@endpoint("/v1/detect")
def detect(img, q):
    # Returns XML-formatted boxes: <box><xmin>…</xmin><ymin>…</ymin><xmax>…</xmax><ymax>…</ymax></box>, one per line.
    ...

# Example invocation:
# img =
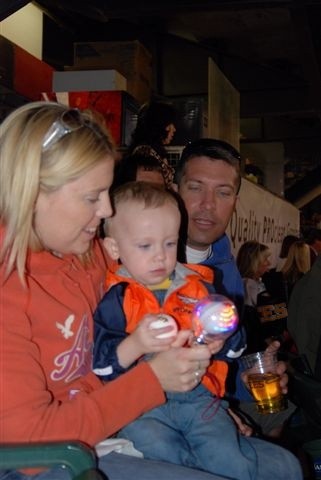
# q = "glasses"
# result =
<box><xmin>43</xmin><ymin>106</ymin><xmax>97</xmax><ymax>150</ymax></box>
<box><xmin>180</xmin><ymin>138</ymin><xmax>243</xmax><ymax>196</ymax></box>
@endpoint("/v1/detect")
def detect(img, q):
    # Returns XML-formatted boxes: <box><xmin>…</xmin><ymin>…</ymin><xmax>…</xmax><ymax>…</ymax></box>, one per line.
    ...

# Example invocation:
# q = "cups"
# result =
<box><xmin>238</xmin><ymin>351</ymin><xmax>288</xmax><ymax>414</ymax></box>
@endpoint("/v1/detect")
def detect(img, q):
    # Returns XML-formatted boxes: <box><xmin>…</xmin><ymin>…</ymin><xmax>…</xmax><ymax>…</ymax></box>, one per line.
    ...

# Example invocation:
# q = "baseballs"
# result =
<box><xmin>152</xmin><ymin>313</ymin><xmax>178</xmax><ymax>344</ymax></box>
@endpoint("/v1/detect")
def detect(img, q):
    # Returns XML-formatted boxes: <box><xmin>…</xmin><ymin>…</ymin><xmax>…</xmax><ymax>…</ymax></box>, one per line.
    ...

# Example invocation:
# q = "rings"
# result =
<box><xmin>196</xmin><ymin>361</ymin><xmax>201</xmax><ymax>370</ymax></box>
<box><xmin>194</xmin><ymin>373</ymin><xmax>201</xmax><ymax>382</ymax></box>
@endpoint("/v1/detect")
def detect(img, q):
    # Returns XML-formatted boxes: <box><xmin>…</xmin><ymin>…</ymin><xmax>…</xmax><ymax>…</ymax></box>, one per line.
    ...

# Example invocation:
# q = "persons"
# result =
<box><xmin>237</xmin><ymin>240</ymin><xmax>271</xmax><ymax>308</ymax></box>
<box><xmin>177</xmin><ymin>138</ymin><xmax>305</xmax><ymax>480</ymax></box>
<box><xmin>0</xmin><ymin>103</ymin><xmax>304</xmax><ymax>480</ymax></box>
<box><xmin>94</xmin><ymin>185</ymin><xmax>258</xmax><ymax>480</ymax></box>
